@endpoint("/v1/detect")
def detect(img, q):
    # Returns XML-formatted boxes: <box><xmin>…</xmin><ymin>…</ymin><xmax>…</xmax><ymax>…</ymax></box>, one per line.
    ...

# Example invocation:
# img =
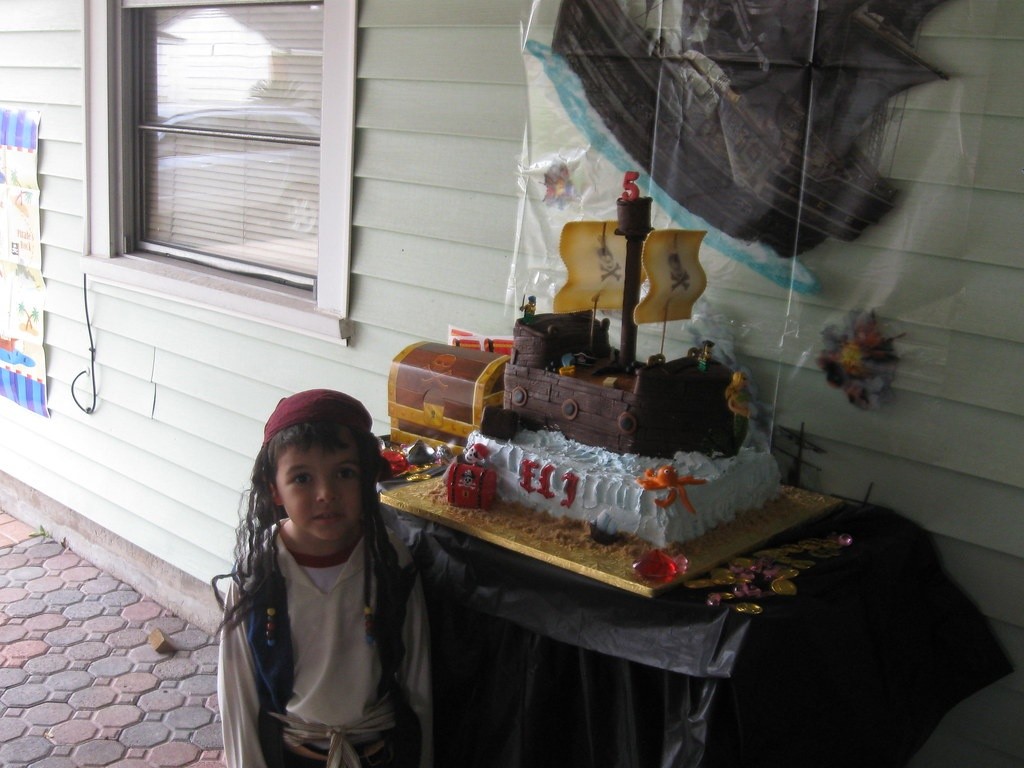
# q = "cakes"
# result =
<box><xmin>443</xmin><ymin>171</ymin><xmax>786</xmax><ymax>554</ymax></box>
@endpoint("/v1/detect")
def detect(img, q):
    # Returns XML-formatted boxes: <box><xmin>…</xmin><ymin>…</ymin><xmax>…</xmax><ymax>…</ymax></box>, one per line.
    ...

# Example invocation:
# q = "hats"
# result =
<box><xmin>265</xmin><ymin>389</ymin><xmax>372</xmax><ymax>445</ymax></box>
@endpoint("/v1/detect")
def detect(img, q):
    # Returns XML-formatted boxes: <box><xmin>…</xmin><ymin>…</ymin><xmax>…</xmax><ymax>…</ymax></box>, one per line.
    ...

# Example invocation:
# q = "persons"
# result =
<box><xmin>218</xmin><ymin>388</ymin><xmax>433</xmax><ymax>767</ymax></box>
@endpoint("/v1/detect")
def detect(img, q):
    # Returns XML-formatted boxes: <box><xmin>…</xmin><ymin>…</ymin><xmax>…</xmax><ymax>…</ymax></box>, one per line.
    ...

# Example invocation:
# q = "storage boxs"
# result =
<box><xmin>387</xmin><ymin>340</ymin><xmax>516</xmax><ymax>460</ymax></box>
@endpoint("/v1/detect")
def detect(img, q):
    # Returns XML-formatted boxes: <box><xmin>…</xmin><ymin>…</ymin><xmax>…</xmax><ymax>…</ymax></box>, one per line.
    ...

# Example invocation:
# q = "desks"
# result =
<box><xmin>381</xmin><ymin>507</ymin><xmax>1014</xmax><ymax>767</ymax></box>
